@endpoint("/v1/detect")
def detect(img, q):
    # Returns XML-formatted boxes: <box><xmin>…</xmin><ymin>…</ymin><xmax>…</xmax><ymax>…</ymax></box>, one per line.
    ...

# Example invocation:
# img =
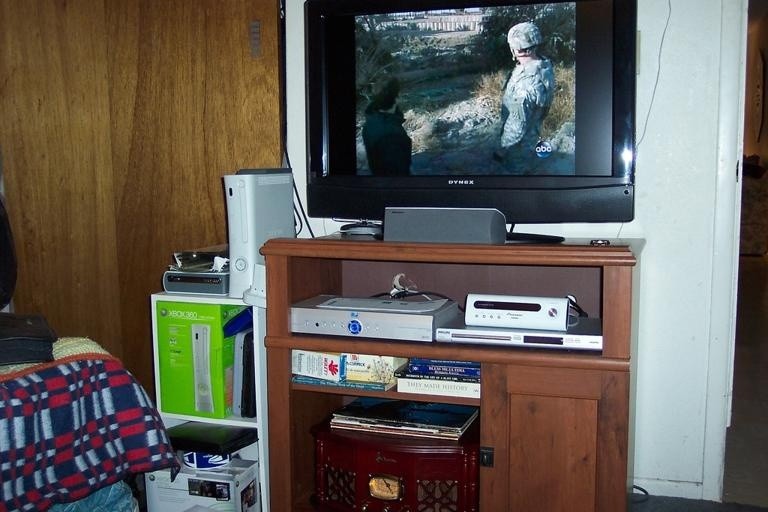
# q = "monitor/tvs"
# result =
<box><xmin>303</xmin><ymin>0</ymin><xmax>637</xmax><ymax>246</ymax></box>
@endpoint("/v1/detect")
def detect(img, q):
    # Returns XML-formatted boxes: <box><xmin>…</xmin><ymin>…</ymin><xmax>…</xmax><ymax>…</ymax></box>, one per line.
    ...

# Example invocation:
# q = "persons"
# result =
<box><xmin>493</xmin><ymin>20</ymin><xmax>554</xmax><ymax>173</ymax></box>
<box><xmin>362</xmin><ymin>75</ymin><xmax>412</xmax><ymax>177</ymax></box>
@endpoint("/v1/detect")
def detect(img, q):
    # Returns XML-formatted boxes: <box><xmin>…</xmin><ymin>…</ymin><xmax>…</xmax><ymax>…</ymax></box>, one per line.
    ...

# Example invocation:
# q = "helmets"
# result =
<box><xmin>506</xmin><ymin>22</ymin><xmax>545</xmax><ymax>51</ymax></box>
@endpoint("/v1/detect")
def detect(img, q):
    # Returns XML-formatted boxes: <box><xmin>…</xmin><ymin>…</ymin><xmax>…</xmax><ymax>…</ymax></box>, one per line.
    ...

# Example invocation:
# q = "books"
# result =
<box><xmin>291</xmin><ymin>357</ymin><xmax>480</xmax><ymax>442</ymax></box>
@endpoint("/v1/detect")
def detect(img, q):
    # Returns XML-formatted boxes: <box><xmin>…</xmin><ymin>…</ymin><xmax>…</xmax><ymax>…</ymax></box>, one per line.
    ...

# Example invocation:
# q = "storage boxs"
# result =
<box><xmin>156</xmin><ymin>302</ymin><xmax>249</xmax><ymax>419</ymax></box>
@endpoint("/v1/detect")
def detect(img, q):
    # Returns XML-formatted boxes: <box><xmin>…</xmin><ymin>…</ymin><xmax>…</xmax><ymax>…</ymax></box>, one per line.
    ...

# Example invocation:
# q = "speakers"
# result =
<box><xmin>383</xmin><ymin>206</ymin><xmax>507</xmax><ymax>247</ymax></box>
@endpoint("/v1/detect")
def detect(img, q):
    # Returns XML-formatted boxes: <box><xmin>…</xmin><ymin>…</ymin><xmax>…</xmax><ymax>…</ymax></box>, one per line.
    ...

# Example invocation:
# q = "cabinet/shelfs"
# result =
<box><xmin>150</xmin><ymin>291</ymin><xmax>269</xmax><ymax>511</ymax></box>
<box><xmin>258</xmin><ymin>233</ymin><xmax>638</xmax><ymax>512</ymax></box>
